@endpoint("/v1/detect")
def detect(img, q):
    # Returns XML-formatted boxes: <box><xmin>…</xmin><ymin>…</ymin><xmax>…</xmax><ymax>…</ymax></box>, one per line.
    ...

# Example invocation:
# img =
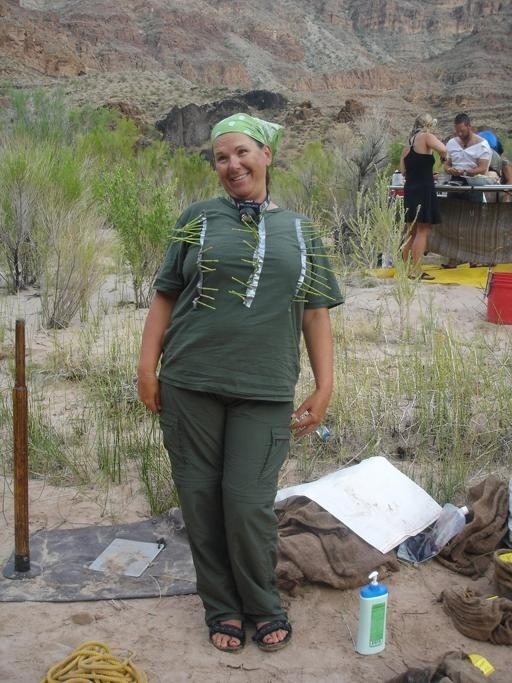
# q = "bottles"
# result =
<box><xmin>391</xmin><ymin>171</ymin><xmax>403</xmax><ymax>186</ymax></box>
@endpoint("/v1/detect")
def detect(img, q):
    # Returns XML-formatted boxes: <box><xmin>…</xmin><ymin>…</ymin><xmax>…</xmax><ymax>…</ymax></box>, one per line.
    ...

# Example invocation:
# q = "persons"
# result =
<box><xmin>440</xmin><ymin>113</ymin><xmax>490</xmax><ymax>269</ymax></box>
<box><xmin>398</xmin><ymin>111</ymin><xmax>447</xmax><ymax>280</ymax></box>
<box><xmin>477</xmin><ymin>129</ymin><xmax>511</xmax><ymax>186</ymax></box>
<box><xmin>134</xmin><ymin>112</ymin><xmax>344</xmax><ymax>655</ymax></box>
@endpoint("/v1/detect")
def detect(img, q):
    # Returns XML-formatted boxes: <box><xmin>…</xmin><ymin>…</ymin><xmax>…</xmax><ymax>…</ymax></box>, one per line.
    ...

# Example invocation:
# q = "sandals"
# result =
<box><xmin>210</xmin><ymin>621</ymin><xmax>292</xmax><ymax>652</ymax></box>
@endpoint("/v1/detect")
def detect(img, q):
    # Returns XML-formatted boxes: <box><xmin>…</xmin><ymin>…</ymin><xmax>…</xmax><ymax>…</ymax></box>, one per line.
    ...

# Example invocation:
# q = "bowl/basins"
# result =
<box><xmin>464</xmin><ymin>176</ymin><xmax>489</xmax><ymax>186</ymax></box>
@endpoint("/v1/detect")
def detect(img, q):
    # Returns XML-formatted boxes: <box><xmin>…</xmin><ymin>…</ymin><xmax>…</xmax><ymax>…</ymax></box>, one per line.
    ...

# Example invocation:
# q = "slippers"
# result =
<box><xmin>409</xmin><ymin>273</ymin><xmax>435</xmax><ymax>280</ymax></box>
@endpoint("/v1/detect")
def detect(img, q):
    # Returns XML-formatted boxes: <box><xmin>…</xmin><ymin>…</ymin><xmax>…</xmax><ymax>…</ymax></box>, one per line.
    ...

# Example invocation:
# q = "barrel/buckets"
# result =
<box><xmin>486</xmin><ymin>273</ymin><xmax>512</xmax><ymax>325</ymax></box>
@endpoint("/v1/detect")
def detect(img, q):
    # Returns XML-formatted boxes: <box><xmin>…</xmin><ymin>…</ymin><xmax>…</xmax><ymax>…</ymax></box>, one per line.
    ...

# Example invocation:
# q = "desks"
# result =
<box><xmin>387</xmin><ymin>186</ymin><xmax>512</xmax><ymax>266</ymax></box>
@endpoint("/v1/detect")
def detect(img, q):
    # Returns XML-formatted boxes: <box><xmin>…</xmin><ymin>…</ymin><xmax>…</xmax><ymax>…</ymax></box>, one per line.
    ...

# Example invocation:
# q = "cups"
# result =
<box><xmin>316</xmin><ymin>425</ymin><xmax>331</xmax><ymax>443</ymax></box>
